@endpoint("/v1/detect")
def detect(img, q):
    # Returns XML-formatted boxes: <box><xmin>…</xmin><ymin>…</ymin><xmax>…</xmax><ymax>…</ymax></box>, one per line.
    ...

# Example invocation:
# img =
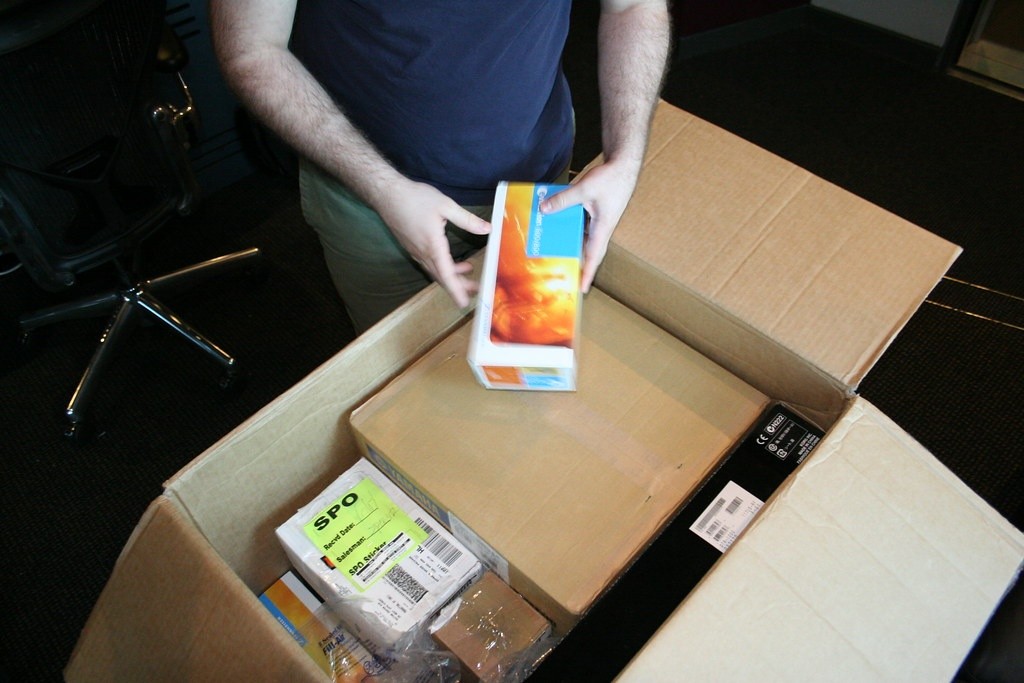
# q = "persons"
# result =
<box><xmin>210</xmin><ymin>0</ymin><xmax>674</xmax><ymax>339</ymax></box>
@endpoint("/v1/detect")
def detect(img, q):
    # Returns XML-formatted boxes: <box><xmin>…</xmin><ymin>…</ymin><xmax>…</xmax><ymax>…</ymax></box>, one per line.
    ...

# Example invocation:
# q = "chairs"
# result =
<box><xmin>0</xmin><ymin>0</ymin><xmax>261</xmax><ymax>446</ymax></box>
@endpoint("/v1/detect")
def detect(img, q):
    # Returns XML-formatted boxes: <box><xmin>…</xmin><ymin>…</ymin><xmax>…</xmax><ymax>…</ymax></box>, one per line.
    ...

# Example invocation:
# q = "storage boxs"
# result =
<box><xmin>61</xmin><ymin>98</ymin><xmax>1024</xmax><ymax>683</ymax></box>
